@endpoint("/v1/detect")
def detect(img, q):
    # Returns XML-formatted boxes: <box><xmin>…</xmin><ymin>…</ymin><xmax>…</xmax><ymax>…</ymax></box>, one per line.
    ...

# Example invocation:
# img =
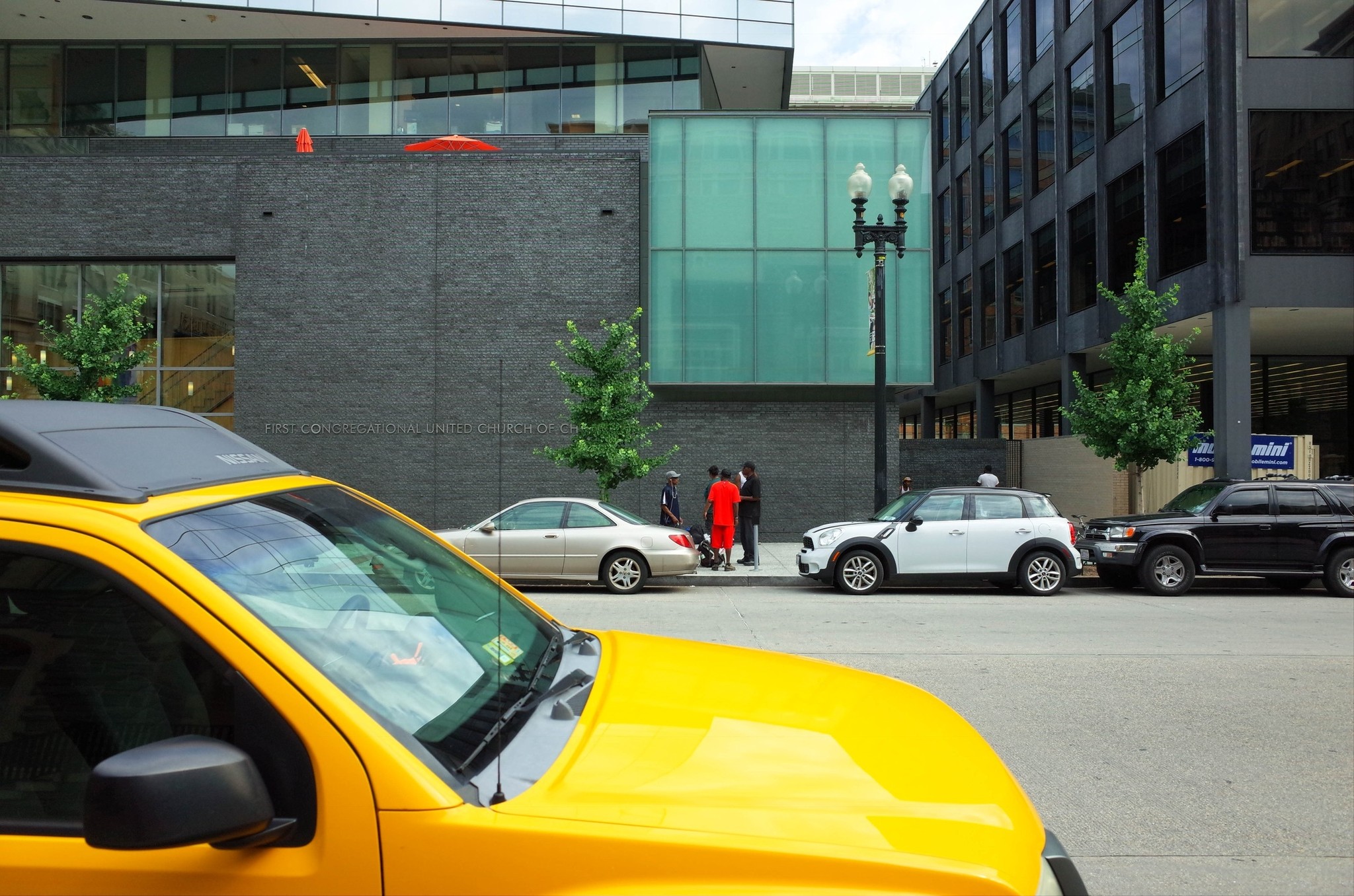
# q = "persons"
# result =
<box><xmin>702</xmin><ymin>460</ymin><xmax>761</xmax><ymax>571</ymax></box>
<box><xmin>900</xmin><ymin>477</ymin><xmax>913</xmax><ymax>495</ymax></box>
<box><xmin>660</xmin><ymin>471</ymin><xmax>683</xmax><ymax>529</ymax></box>
<box><xmin>976</xmin><ymin>465</ymin><xmax>1000</xmax><ymax>487</ymax></box>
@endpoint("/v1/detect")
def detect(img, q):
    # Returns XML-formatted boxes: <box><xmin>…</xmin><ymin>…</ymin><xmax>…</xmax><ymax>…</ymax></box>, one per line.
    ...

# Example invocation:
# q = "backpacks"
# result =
<box><xmin>689</xmin><ymin>524</ymin><xmax>705</xmax><ymax>544</ymax></box>
<box><xmin>696</xmin><ymin>540</ymin><xmax>725</xmax><ymax>567</ymax></box>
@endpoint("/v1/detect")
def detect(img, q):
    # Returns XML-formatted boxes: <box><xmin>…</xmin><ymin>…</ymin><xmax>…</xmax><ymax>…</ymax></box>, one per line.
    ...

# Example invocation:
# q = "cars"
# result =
<box><xmin>371</xmin><ymin>499</ymin><xmax>701</xmax><ymax>592</ymax></box>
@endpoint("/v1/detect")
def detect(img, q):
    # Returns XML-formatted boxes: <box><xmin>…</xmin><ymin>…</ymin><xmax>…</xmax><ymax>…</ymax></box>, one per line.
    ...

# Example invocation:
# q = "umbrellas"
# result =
<box><xmin>296</xmin><ymin>127</ymin><xmax>313</xmax><ymax>152</ymax></box>
<box><xmin>403</xmin><ymin>135</ymin><xmax>503</xmax><ymax>151</ymax></box>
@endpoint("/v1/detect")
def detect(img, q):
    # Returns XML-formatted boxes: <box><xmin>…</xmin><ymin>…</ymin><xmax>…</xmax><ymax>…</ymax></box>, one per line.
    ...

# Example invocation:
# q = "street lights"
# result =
<box><xmin>844</xmin><ymin>162</ymin><xmax>914</xmax><ymax>514</ymax></box>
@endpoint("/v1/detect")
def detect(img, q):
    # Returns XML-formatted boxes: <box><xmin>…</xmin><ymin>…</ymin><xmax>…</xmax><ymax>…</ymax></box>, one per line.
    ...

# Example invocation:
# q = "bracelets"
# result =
<box><xmin>735</xmin><ymin>517</ymin><xmax>738</xmax><ymax>519</ymax></box>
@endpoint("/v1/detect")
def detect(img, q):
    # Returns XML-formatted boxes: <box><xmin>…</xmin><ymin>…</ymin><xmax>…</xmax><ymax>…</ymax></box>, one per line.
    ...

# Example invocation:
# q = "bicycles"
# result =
<box><xmin>1071</xmin><ymin>514</ymin><xmax>1088</xmax><ymax>544</ymax></box>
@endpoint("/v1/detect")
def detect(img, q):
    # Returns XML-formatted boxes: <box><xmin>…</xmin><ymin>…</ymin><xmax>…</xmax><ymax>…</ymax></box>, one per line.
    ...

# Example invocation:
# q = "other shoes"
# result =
<box><xmin>725</xmin><ymin>564</ymin><xmax>736</xmax><ymax>571</ymax></box>
<box><xmin>743</xmin><ymin>560</ymin><xmax>760</xmax><ymax>565</ymax></box>
<box><xmin>712</xmin><ymin>564</ymin><xmax>718</xmax><ymax>571</ymax></box>
<box><xmin>737</xmin><ymin>557</ymin><xmax>745</xmax><ymax>563</ymax></box>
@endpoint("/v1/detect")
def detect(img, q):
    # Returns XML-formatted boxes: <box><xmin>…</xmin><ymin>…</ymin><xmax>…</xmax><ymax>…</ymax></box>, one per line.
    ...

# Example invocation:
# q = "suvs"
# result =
<box><xmin>1</xmin><ymin>396</ymin><xmax>1094</xmax><ymax>896</ymax></box>
<box><xmin>796</xmin><ymin>485</ymin><xmax>1083</xmax><ymax>596</ymax></box>
<box><xmin>1072</xmin><ymin>472</ymin><xmax>1354</xmax><ymax>597</ymax></box>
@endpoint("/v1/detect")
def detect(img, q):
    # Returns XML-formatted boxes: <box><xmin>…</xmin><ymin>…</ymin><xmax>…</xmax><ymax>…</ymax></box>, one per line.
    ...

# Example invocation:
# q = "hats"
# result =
<box><xmin>904</xmin><ymin>477</ymin><xmax>912</xmax><ymax>481</ymax></box>
<box><xmin>666</xmin><ymin>471</ymin><xmax>681</xmax><ymax>479</ymax></box>
<box><xmin>740</xmin><ymin>461</ymin><xmax>755</xmax><ymax>470</ymax></box>
<box><xmin>707</xmin><ymin>465</ymin><xmax>719</xmax><ymax>473</ymax></box>
<box><xmin>719</xmin><ymin>468</ymin><xmax>731</xmax><ymax>478</ymax></box>
